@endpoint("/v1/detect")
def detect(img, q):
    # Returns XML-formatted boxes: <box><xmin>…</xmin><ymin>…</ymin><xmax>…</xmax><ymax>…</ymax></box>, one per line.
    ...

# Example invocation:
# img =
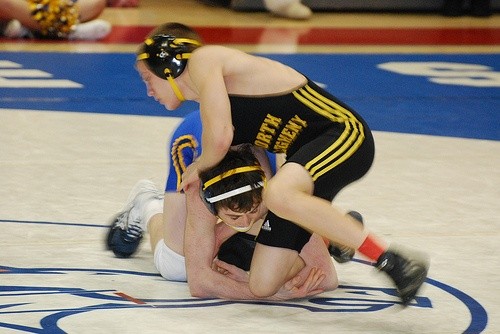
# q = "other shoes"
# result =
<box><xmin>264</xmin><ymin>0</ymin><xmax>312</xmax><ymax>19</ymax></box>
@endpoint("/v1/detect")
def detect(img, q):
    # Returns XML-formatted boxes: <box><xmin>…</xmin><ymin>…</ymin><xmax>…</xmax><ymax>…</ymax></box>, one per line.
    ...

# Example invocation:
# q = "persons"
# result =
<box><xmin>0</xmin><ymin>0</ymin><xmax>112</xmax><ymax>41</ymax></box>
<box><xmin>106</xmin><ymin>109</ymin><xmax>338</xmax><ymax>300</ymax></box>
<box><xmin>135</xmin><ymin>23</ymin><xmax>429</xmax><ymax>308</ymax></box>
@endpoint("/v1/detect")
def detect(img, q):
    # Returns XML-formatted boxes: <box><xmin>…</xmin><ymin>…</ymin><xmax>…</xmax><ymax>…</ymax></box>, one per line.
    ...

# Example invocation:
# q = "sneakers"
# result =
<box><xmin>108</xmin><ymin>181</ymin><xmax>159</xmax><ymax>258</ymax></box>
<box><xmin>64</xmin><ymin>20</ymin><xmax>111</xmax><ymax>40</ymax></box>
<box><xmin>378</xmin><ymin>246</ymin><xmax>431</xmax><ymax>296</ymax></box>
<box><xmin>327</xmin><ymin>211</ymin><xmax>363</xmax><ymax>264</ymax></box>
<box><xmin>4</xmin><ymin>19</ymin><xmax>22</xmax><ymax>38</ymax></box>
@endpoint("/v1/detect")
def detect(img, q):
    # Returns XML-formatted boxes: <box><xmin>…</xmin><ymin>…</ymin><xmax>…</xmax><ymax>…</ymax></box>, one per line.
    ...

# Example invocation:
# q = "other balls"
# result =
<box><xmin>217</xmin><ymin>232</ymin><xmax>257</xmax><ymax>271</ymax></box>
